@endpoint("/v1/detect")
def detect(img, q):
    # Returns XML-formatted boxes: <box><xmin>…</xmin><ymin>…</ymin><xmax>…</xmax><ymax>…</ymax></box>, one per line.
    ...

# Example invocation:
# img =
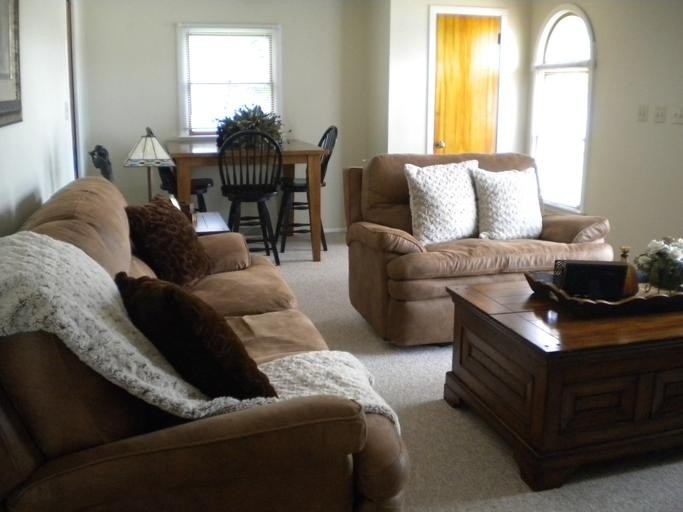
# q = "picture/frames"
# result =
<box><xmin>0</xmin><ymin>0</ymin><xmax>23</xmax><ymax>128</ymax></box>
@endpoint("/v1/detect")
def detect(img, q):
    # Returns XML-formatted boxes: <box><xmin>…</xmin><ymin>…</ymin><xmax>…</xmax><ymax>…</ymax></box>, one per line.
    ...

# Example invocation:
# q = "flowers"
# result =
<box><xmin>632</xmin><ymin>236</ymin><xmax>683</xmax><ymax>271</ymax></box>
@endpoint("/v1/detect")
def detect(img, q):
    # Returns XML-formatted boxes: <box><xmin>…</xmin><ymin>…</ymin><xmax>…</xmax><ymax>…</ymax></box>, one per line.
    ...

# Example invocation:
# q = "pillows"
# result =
<box><xmin>360</xmin><ymin>155</ymin><xmax>547</xmax><ymax>249</ymax></box>
<box><xmin>112</xmin><ymin>195</ymin><xmax>280</xmax><ymax>405</ymax></box>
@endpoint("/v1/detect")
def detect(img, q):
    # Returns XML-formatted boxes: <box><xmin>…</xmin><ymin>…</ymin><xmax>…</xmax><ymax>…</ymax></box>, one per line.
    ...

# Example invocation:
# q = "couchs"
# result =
<box><xmin>2</xmin><ymin>175</ymin><xmax>409</xmax><ymax>512</ymax></box>
<box><xmin>341</xmin><ymin>165</ymin><xmax>615</xmax><ymax>349</ymax></box>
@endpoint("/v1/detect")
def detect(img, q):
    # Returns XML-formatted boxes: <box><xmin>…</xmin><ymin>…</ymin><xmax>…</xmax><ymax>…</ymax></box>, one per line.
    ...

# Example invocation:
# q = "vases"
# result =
<box><xmin>641</xmin><ymin>265</ymin><xmax>681</xmax><ymax>297</ymax></box>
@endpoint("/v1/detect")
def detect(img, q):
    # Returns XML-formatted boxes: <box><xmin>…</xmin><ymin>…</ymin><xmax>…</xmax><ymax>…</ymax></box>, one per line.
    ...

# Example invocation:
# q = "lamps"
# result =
<box><xmin>122</xmin><ymin>135</ymin><xmax>176</xmax><ymax>202</ymax></box>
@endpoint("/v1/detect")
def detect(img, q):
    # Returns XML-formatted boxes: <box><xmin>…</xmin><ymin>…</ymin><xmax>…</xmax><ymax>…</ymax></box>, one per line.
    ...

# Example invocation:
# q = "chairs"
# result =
<box><xmin>146</xmin><ymin>126</ymin><xmax>337</xmax><ymax>266</ymax></box>
